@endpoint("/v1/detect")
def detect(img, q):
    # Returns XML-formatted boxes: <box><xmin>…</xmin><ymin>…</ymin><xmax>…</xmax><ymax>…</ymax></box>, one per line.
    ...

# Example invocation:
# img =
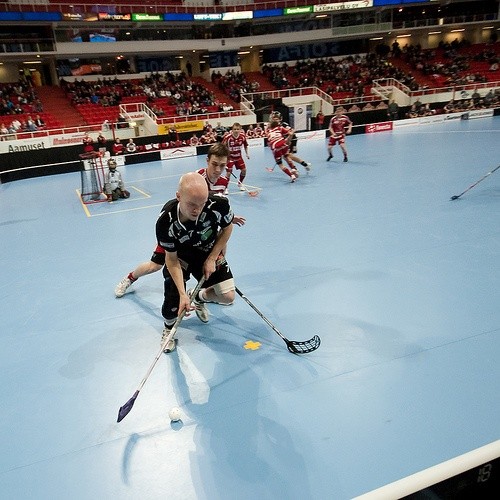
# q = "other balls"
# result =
<box><xmin>169</xmin><ymin>408</ymin><xmax>180</xmax><ymax>421</ymax></box>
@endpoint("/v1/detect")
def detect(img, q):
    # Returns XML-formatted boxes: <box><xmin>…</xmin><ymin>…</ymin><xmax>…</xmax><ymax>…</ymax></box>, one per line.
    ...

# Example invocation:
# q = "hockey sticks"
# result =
<box><xmin>224</xmin><ymin>165</ymin><xmax>259</xmax><ymax>198</ymax></box>
<box><xmin>117</xmin><ymin>274</ymin><xmax>206</xmax><ymax>423</ymax></box>
<box><xmin>235</xmin><ymin>286</ymin><xmax>321</xmax><ymax>354</ymax></box>
<box><xmin>266</xmin><ymin>162</ymin><xmax>277</xmax><ymax>172</ymax></box>
<box><xmin>450</xmin><ymin>165</ymin><xmax>500</xmax><ymax>200</ymax></box>
<box><xmin>292</xmin><ymin>132</ymin><xmax>316</xmax><ymax>140</ymax></box>
<box><xmin>330</xmin><ymin>132</ymin><xmax>346</xmax><ymax>139</ymax></box>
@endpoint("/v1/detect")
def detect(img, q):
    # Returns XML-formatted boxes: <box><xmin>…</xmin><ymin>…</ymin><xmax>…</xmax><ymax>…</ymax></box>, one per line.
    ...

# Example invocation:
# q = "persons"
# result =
<box><xmin>102</xmin><ymin>159</ymin><xmax>130</xmax><ymax>202</ymax></box>
<box><xmin>271</xmin><ymin>111</ymin><xmax>311</xmax><ymax>174</ymax></box>
<box><xmin>326</xmin><ymin>106</ymin><xmax>352</xmax><ymax>162</ymax></box>
<box><xmin>265</xmin><ymin>121</ymin><xmax>299</xmax><ymax>183</ymax></box>
<box><xmin>154</xmin><ymin>172</ymin><xmax>236</xmax><ymax>353</ymax></box>
<box><xmin>97</xmin><ymin>131</ymin><xmax>106</xmax><ymax>147</ymax></box>
<box><xmin>113</xmin><ymin>144</ymin><xmax>248</xmax><ymax>297</ymax></box>
<box><xmin>83</xmin><ymin>132</ymin><xmax>93</xmax><ymax>152</ymax></box>
<box><xmin>0</xmin><ymin>63</ymin><xmax>45</xmax><ymax>135</ymax></box>
<box><xmin>221</xmin><ymin>122</ymin><xmax>250</xmax><ymax>196</ymax></box>
<box><xmin>62</xmin><ymin>38</ymin><xmax>500</xmax><ymax>164</ymax></box>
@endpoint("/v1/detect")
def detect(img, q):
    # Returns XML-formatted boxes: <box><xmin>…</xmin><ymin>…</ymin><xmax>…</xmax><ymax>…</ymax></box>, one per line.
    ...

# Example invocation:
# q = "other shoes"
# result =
<box><xmin>344</xmin><ymin>156</ymin><xmax>347</xmax><ymax>162</ymax></box>
<box><xmin>237</xmin><ymin>183</ymin><xmax>245</xmax><ymax>191</ymax></box>
<box><xmin>306</xmin><ymin>163</ymin><xmax>311</xmax><ymax>171</ymax></box>
<box><xmin>224</xmin><ymin>188</ymin><xmax>228</xmax><ymax>195</ymax></box>
<box><xmin>292</xmin><ymin>169</ymin><xmax>299</xmax><ymax>177</ymax></box>
<box><xmin>291</xmin><ymin>175</ymin><xmax>296</xmax><ymax>182</ymax></box>
<box><xmin>327</xmin><ymin>155</ymin><xmax>333</xmax><ymax>161</ymax></box>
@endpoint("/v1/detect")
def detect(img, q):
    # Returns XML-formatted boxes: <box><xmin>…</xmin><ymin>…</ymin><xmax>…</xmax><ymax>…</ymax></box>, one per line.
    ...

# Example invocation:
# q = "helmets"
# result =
<box><xmin>272</xmin><ymin>111</ymin><xmax>281</xmax><ymax>118</ymax></box>
<box><xmin>108</xmin><ymin>159</ymin><xmax>116</xmax><ymax>170</ymax></box>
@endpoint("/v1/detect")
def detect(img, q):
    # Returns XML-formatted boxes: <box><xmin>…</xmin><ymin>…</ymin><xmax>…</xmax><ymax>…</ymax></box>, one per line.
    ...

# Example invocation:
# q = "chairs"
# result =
<box><xmin>214</xmin><ymin>42</ymin><xmax>500</xmax><ymax>102</ymax></box>
<box><xmin>135</xmin><ymin>139</ymin><xmax>190</xmax><ymax>150</ymax></box>
<box><xmin>0</xmin><ymin>82</ymin><xmax>68</xmax><ymax>141</ymax></box>
<box><xmin>62</xmin><ymin>75</ymin><xmax>241</xmax><ymax>130</ymax></box>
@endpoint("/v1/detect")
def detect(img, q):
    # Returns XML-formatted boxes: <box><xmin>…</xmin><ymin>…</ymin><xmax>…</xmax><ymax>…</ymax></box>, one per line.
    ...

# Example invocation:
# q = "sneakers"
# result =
<box><xmin>115</xmin><ymin>273</ymin><xmax>133</xmax><ymax>298</ymax></box>
<box><xmin>161</xmin><ymin>327</ymin><xmax>176</xmax><ymax>352</ymax></box>
<box><xmin>188</xmin><ymin>287</ymin><xmax>209</xmax><ymax>323</ymax></box>
